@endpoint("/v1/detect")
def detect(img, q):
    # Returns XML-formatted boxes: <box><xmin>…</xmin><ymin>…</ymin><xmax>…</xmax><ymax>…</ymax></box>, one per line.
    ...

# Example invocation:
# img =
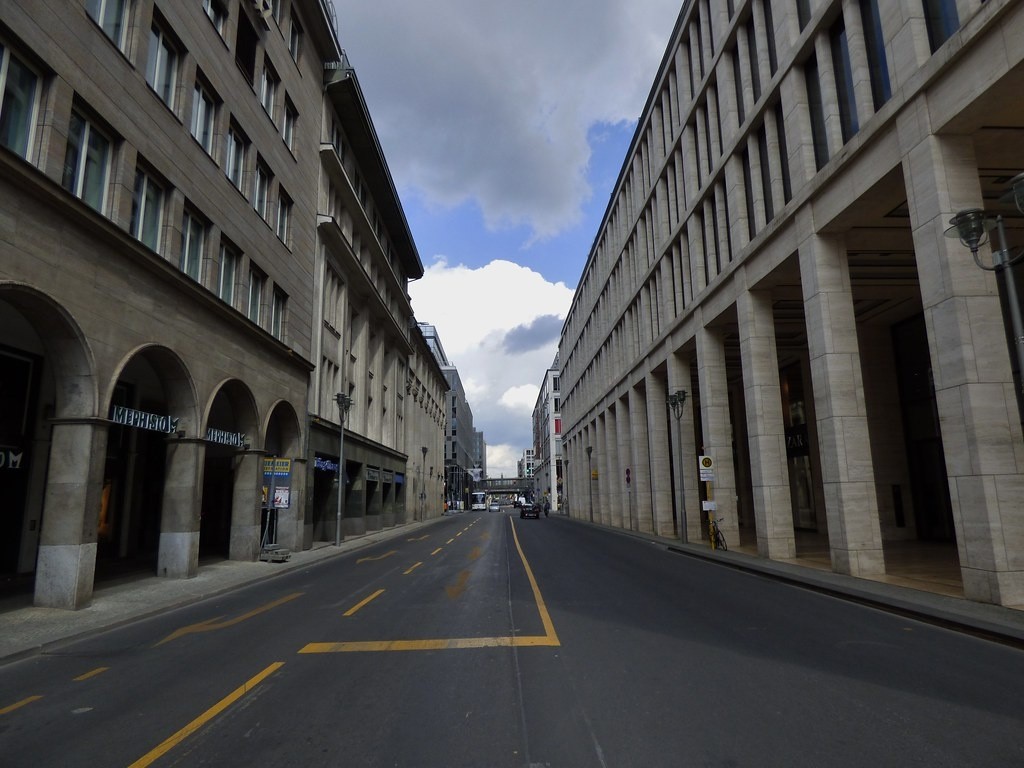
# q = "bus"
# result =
<box><xmin>471</xmin><ymin>492</ymin><xmax>487</xmax><ymax>510</ymax></box>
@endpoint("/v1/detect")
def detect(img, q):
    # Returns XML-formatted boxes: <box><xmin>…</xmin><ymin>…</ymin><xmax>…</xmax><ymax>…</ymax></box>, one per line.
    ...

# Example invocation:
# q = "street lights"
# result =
<box><xmin>584</xmin><ymin>443</ymin><xmax>595</xmax><ymax>522</ymax></box>
<box><xmin>330</xmin><ymin>389</ymin><xmax>353</xmax><ymax>548</ymax></box>
<box><xmin>419</xmin><ymin>443</ymin><xmax>429</xmax><ymax>524</ymax></box>
<box><xmin>666</xmin><ymin>387</ymin><xmax>690</xmax><ymax>545</ymax></box>
<box><xmin>563</xmin><ymin>458</ymin><xmax>571</xmax><ymax>517</ymax></box>
<box><xmin>941</xmin><ymin>202</ymin><xmax>1023</xmax><ymax>381</ymax></box>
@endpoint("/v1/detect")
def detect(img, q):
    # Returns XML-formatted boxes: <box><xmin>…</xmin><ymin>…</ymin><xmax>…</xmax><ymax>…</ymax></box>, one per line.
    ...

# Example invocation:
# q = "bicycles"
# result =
<box><xmin>703</xmin><ymin>515</ymin><xmax>729</xmax><ymax>551</ymax></box>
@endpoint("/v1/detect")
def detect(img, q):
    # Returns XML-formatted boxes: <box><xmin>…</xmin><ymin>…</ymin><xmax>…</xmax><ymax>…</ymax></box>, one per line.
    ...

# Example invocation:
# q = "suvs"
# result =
<box><xmin>513</xmin><ymin>501</ymin><xmax>522</xmax><ymax>508</ymax></box>
<box><xmin>519</xmin><ymin>504</ymin><xmax>540</xmax><ymax>518</ymax></box>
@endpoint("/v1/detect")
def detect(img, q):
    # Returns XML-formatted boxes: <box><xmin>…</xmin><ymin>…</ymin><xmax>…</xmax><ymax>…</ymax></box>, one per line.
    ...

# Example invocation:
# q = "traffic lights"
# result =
<box><xmin>526</xmin><ymin>469</ymin><xmax>530</xmax><ymax>474</ymax></box>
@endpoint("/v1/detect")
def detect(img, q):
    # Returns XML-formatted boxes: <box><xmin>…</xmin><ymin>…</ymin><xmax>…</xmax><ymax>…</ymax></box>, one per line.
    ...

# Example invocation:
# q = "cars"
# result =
<box><xmin>488</xmin><ymin>503</ymin><xmax>500</xmax><ymax>512</ymax></box>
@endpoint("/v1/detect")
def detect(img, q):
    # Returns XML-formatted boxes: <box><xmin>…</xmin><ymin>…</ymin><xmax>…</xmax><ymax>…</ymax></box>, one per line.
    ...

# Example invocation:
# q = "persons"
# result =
<box><xmin>545</xmin><ymin>500</ymin><xmax>550</xmax><ymax>509</ymax></box>
